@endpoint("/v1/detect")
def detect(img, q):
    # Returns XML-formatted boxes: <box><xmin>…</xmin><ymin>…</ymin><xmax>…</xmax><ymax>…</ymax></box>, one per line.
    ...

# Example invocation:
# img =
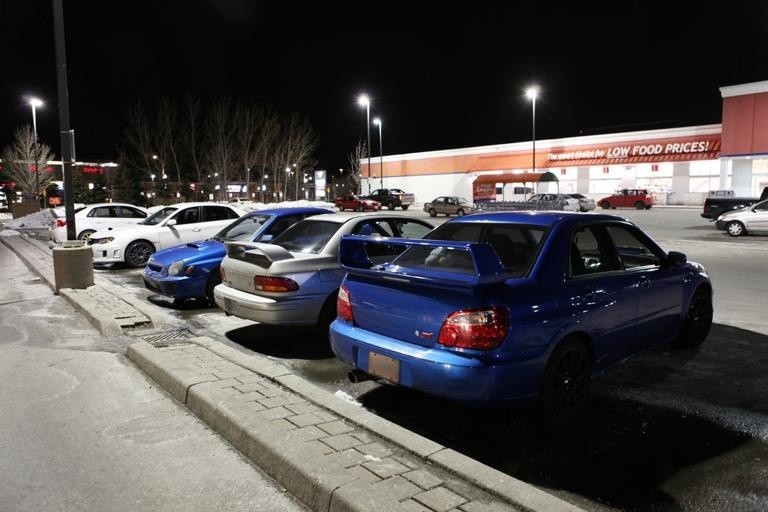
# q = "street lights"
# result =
<box><xmin>138</xmin><ymin>154</ymin><xmax>302</xmax><ymax>203</ymax></box>
<box><xmin>354</xmin><ymin>92</ymin><xmax>372</xmax><ymax>195</ymax></box>
<box><xmin>524</xmin><ymin>86</ymin><xmax>538</xmax><ymax>171</ymax></box>
<box><xmin>370</xmin><ymin>116</ymin><xmax>383</xmax><ymax>189</ymax></box>
<box><xmin>26</xmin><ymin>97</ymin><xmax>48</xmax><ymax>210</ymax></box>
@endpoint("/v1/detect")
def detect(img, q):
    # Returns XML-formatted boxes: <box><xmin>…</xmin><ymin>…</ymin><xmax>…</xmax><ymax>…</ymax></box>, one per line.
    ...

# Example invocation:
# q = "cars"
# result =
<box><xmin>324</xmin><ymin>205</ymin><xmax>717</xmax><ymax>424</ymax></box>
